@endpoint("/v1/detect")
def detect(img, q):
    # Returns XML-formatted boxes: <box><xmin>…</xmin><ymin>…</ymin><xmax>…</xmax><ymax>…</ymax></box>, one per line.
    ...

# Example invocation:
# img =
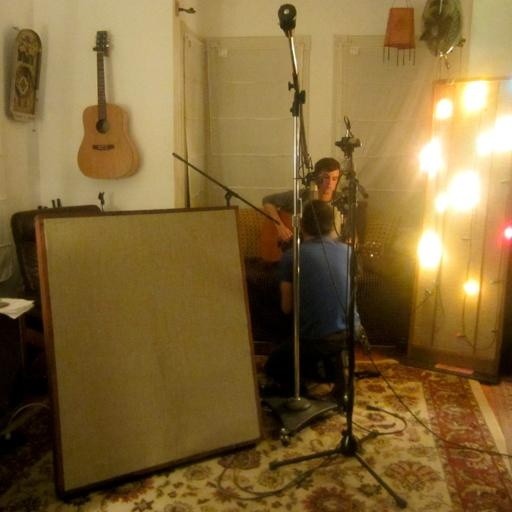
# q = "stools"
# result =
<box><xmin>323</xmin><ymin>331</ymin><xmax>348</xmax><ymax>408</ymax></box>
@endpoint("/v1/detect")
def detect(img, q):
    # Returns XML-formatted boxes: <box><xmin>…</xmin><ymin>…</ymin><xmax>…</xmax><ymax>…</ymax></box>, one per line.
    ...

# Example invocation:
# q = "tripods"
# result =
<box><xmin>267</xmin><ymin>159</ymin><xmax>410</xmax><ymax>509</ymax></box>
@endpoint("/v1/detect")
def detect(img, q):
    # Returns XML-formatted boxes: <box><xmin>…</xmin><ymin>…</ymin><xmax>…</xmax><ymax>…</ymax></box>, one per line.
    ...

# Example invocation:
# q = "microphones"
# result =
<box><xmin>278</xmin><ymin>4</ymin><xmax>297</xmax><ymax>37</ymax></box>
<box><xmin>335</xmin><ymin>116</ymin><xmax>363</xmax><ymax>158</ymax></box>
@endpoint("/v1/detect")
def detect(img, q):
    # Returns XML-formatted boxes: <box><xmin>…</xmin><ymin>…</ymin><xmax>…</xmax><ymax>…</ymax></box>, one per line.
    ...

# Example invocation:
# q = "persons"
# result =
<box><xmin>262</xmin><ymin>157</ymin><xmax>353</xmax><ymax>250</ymax></box>
<box><xmin>259</xmin><ymin>202</ymin><xmax>355</xmax><ymax>397</ymax></box>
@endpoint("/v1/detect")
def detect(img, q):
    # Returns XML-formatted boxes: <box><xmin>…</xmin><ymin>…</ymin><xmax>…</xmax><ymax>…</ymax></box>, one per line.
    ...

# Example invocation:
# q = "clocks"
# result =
<box><xmin>8</xmin><ymin>29</ymin><xmax>43</xmax><ymax>122</ymax></box>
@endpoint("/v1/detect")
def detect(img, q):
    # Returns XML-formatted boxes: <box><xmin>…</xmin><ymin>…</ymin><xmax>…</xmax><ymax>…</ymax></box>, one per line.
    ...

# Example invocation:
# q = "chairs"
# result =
<box><xmin>13</xmin><ymin>204</ymin><xmax>101</xmax><ymax>333</ymax></box>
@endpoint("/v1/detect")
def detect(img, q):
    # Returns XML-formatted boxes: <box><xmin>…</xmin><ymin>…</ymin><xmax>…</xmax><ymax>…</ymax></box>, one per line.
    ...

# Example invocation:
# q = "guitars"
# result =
<box><xmin>259</xmin><ymin>192</ymin><xmax>350</xmax><ymax>261</ymax></box>
<box><xmin>77</xmin><ymin>30</ymin><xmax>140</xmax><ymax>180</ymax></box>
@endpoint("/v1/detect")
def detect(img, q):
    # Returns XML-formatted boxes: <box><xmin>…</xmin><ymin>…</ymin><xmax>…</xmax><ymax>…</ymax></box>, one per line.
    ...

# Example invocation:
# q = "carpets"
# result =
<box><xmin>0</xmin><ymin>354</ymin><xmax>512</xmax><ymax>512</ymax></box>
